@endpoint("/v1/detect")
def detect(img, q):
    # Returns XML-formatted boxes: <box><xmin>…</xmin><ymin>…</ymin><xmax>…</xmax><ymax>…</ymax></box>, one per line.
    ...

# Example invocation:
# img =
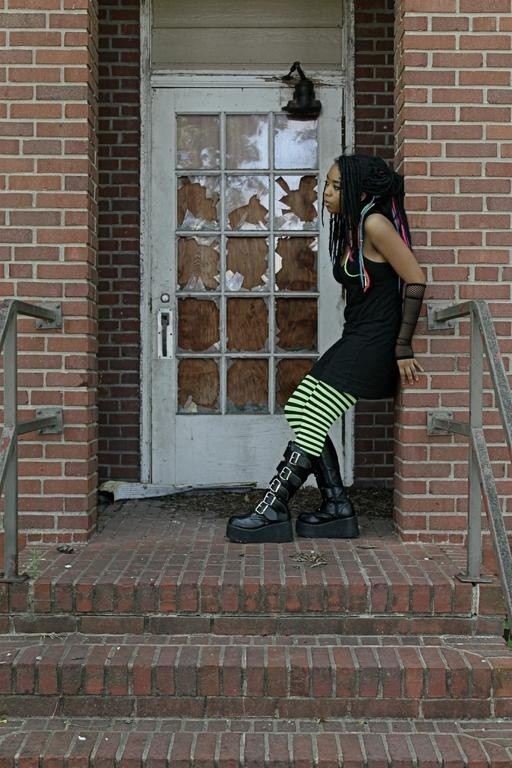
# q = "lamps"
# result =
<box><xmin>272</xmin><ymin>61</ymin><xmax>323</xmax><ymax>121</ymax></box>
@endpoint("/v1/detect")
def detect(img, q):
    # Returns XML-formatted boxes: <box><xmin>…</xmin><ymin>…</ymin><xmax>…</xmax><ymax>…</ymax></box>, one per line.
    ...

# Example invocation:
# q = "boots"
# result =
<box><xmin>222</xmin><ymin>440</ymin><xmax>320</xmax><ymax>544</ymax></box>
<box><xmin>295</xmin><ymin>435</ymin><xmax>361</xmax><ymax>539</ymax></box>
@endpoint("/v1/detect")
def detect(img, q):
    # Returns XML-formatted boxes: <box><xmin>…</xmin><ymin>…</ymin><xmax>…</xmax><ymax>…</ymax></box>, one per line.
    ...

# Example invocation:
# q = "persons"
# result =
<box><xmin>226</xmin><ymin>152</ymin><xmax>428</xmax><ymax>544</ymax></box>
